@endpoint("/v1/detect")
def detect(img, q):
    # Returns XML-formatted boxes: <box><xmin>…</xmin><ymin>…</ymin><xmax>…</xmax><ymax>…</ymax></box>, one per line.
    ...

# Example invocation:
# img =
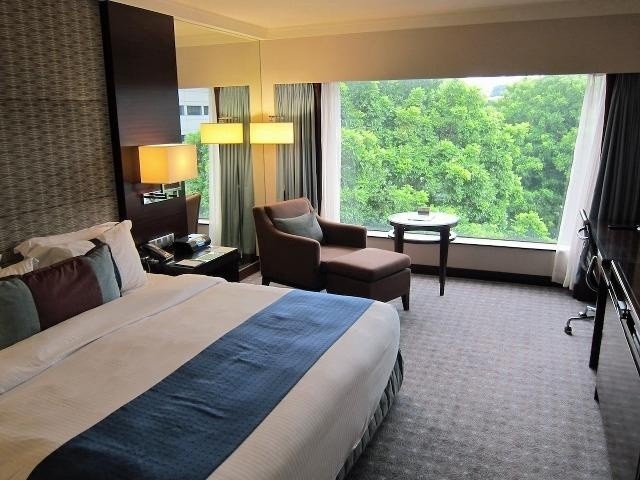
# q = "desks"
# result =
<box><xmin>588</xmin><ymin>216</ymin><xmax>640</xmax><ymax>373</ymax></box>
<box><xmin>383</xmin><ymin>208</ymin><xmax>461</xmax><ymax>299</ymax></box>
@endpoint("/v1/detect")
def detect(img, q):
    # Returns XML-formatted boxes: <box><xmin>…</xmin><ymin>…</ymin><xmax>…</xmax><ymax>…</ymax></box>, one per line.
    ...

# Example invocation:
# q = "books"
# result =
<box><xmin>175</xmin><ymin>259</ymin><xmax>206</xmax><ymax>267</ymax></box>
<box><xmin>407</xmin><ymin>211</ymin><xmax>436</xmax><ymax>222</ymax></box>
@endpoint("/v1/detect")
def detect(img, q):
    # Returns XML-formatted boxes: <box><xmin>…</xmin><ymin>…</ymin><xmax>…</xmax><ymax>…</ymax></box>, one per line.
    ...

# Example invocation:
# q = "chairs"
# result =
<box><xmin>560</xmin><ymin>208</ymin><xmax>597</xmax><ymax>340</ymax></box>
<box><xmin>252</xmin><ymin>197</ymin><xmax>370</xmax><ymax>295</ymax></box>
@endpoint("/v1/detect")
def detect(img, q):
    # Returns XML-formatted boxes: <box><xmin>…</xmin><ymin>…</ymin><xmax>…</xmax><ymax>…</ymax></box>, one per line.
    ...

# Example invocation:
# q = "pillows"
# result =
<box><xmin>0</xmin><ymin>217</ymin><xmax>153</xmax><ymax>354</ymax></box>
<box><xmin>271</xmin><ymin>208</ymin><xmax>323</xmax><ymax>247</ymax></box>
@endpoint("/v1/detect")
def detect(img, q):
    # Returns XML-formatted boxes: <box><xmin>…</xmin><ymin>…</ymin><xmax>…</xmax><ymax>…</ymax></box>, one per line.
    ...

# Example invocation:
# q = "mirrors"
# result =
<box><xmin>170</xmin><ymin>13</ymin><xmax>266</xmax><ymax>272</ymax></box>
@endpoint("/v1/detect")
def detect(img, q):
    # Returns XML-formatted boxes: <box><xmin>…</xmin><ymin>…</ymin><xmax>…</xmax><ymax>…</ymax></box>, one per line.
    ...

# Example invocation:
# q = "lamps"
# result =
<box><xmin>137</xmin><ymin>143</ymin><xmax>199</xmax><ymax>206</ymax></box>
<box><xmin>248</xmin><ymin>113</ymin><xmax>294</xmax><ymax>146</ymax></box>
<box><xmin>199</xmin><ymin>113</ymin><xmax>245</xmax><ymax>145</ymax></box>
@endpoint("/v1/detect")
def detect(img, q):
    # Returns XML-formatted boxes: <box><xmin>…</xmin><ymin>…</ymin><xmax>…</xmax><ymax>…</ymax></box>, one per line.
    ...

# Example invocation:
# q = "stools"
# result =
<box><xmin>324</xmin><ymin>244</ymin><xmax>413</xmax><ymax>314</ymax></box>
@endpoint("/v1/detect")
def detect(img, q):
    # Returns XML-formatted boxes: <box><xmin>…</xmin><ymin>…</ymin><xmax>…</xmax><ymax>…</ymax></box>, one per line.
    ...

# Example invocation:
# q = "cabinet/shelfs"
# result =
<box><xmin>593</xmin><ymin>257</ymin><xmax>639</xmax><ymax>480</ymax></box>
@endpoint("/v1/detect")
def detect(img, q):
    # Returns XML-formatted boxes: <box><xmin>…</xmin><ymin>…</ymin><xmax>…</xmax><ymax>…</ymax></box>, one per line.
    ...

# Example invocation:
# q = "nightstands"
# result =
<box><xmin>141</xmin><ymin>241</ymin><xmax>243</xmax><ymax>284</ymax></box>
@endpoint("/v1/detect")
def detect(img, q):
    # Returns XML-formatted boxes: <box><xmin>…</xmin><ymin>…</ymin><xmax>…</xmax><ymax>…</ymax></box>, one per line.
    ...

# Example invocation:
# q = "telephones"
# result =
<box><xmin>139</xmin><ymin>243</ymin><xmax>174</xmax><ymax>264</ymax></box>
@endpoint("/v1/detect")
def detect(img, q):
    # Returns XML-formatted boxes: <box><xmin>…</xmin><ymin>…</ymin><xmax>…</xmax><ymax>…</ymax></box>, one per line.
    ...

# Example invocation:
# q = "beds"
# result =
<box><xmin>0</xmin><ymin>274</ymin><xmax>412</xmax><ymax>478</ymax></box>
